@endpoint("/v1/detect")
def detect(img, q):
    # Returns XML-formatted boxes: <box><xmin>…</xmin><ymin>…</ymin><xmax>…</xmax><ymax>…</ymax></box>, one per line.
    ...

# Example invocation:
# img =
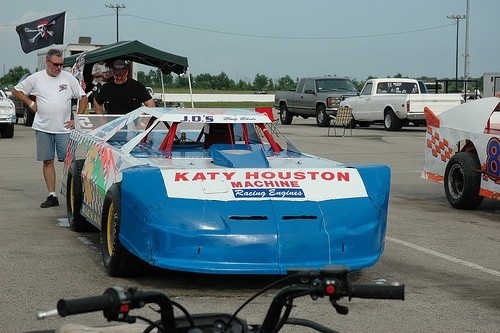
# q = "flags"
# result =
<box><xmin>16</xmin><ymin>11</ymin><xmax>66</xmax><ymax>54</ymax></box>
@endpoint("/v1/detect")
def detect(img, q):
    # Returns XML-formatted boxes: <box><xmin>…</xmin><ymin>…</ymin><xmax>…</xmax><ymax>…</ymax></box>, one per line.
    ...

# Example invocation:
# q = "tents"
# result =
<box><xmin>63</xmin><ymin>39</ymin><xmax>194</xmax><ymax>108</ymax></box>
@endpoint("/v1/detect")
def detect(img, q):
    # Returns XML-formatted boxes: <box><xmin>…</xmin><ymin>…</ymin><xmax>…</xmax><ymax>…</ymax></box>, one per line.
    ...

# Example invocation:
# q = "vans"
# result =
<box><xmin>424</xmin><ymin>83</ymin><xmax>445</xmax><ymax>94</ymax></box>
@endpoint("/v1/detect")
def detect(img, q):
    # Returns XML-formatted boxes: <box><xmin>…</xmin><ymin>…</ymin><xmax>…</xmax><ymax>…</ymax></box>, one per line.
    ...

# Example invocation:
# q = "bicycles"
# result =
<box><xmin>36</xmin><ymin>274</ymin><xmax>406</xmax><ymax>333</ymax></box>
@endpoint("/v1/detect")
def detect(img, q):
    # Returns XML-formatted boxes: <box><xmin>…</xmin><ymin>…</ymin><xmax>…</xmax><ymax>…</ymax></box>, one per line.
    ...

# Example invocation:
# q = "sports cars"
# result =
<box><xmin>59</xmin><ymin>103</ymin><xmax>392</xmax><ymax>281</ymax></box>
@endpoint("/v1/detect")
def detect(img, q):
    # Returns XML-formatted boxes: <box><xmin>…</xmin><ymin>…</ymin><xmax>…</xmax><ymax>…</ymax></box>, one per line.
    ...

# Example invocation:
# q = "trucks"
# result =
<box><xmin>37</xmin><ymin>43</ymin><xmax>137</xmax><ymax>119</ymax></box>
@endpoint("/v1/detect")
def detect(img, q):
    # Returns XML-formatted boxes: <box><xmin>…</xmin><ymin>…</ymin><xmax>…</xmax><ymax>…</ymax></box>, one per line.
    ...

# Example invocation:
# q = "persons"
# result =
<box><xmin>94</xmin><ymin>58</ymin><xmax>155</xmax><ymax>130</ymax></box>
<box><xmin>11</xmin><ymin>48</ymin><xmax>89</xmax><ymax>208</ymax></box>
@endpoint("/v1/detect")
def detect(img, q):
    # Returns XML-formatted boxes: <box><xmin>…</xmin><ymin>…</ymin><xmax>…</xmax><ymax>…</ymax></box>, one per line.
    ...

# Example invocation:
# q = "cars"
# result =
<box><xmin>0</xmin><ymin>86</ymin><xmax>18</xmax><ymax>139</ymax></box>
<box><xmin>421</xmin><ymin>94</ymin><xmax>500</xmax><ymax>210</ymax></box>
<box><xmin>146</xmin><ymin>86</ymin><xmax>162</xmax><ymax>103</ymax></box>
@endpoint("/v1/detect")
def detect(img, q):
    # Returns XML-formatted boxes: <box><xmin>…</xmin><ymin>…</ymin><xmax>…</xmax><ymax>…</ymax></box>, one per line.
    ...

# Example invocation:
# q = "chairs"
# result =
<box><xmin>328</xmin><ymin>107</ymin><xmax>352</xmax><ymax>137</ymax></box>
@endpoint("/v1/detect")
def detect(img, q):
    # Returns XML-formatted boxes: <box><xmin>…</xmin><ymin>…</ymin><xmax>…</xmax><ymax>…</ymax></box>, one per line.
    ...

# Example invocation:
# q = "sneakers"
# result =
<box><xmin>40</xmin><ymin>195</ymin><xmax>59</xmax><ymax>208</ymax></box>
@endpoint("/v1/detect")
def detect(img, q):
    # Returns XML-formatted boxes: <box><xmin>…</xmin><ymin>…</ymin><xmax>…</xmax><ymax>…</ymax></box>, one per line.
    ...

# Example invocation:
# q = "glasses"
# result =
<box><xmin>48</xmin><ymin>59</ymin><xmax>63</xmax><ymax>66</ymax></box>
<box><xmin>113</xmin><ymin>69</ymin><xmax>127</xmax><ymax>76</ymax></box>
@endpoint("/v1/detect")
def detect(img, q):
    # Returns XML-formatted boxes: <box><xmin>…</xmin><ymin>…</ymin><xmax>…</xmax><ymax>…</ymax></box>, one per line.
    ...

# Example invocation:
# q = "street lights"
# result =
<box><xmin>105</xmin><ymin>3</ymin><xmax>126</xmax><ymax>42</ymax></box>
<box><xmin>447</xmin><ymin>14</ymin><xmax>465</xmax><ymax>94</ymax></box>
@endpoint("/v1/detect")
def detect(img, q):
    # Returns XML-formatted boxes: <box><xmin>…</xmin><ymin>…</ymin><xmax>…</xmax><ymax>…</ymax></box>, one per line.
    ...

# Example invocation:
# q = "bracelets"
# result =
<box><xmin>29</xmin><ymin>101</ymin><xmax>34</xmax><ymax>108</ymax></box>
<box><xmin>140</xmin><ymin>122</ymin><xmax>146</xmax><ymax>127</ymax></box>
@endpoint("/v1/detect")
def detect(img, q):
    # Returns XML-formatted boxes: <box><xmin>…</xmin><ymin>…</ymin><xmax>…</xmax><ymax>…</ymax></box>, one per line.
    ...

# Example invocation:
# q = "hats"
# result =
<box><xmin>113</xmin><ymin>59</ymin><xmax>125</xmax><ymax>69</ymax></box>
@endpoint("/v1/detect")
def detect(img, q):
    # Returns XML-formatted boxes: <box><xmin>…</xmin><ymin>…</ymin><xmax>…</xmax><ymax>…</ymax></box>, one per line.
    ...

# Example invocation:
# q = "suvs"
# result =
<box><xmin>8</xmin><ymin>73</ymin><xmax>37</xmax><ymax>126</ymax></box>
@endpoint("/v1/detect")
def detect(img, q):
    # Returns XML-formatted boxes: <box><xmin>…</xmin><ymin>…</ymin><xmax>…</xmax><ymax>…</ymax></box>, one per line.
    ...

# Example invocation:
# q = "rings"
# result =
<box><xmin>70</xmin><ymin>124</ymin><xmax>73</xmax><ymax>127</ymax></box>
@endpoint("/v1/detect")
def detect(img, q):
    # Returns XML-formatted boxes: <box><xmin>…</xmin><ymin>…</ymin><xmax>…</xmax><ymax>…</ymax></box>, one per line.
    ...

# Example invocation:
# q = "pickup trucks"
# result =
<box><xmin>273</xmin><ymin>76</ymin><xmax>360</xmax><ymax>127</ymax></box>
<box><xmin>340</xmin><ymin>78</ymin><xmax>465</xmax><ymax>132</ymax></box>
